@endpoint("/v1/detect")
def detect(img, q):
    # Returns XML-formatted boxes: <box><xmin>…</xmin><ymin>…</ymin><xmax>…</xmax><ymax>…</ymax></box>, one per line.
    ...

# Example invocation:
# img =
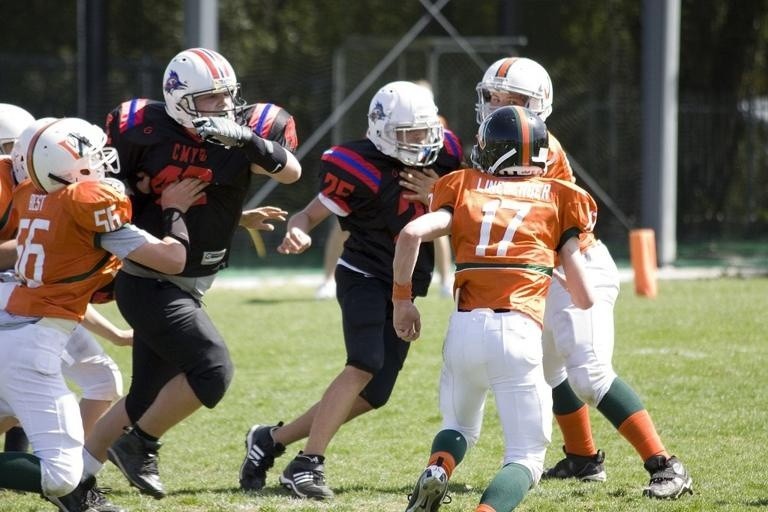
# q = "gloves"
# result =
<box><xmin>192</xmin><ymin>116</ymin><xmax>251</xmax><ymax>149</ymax></box>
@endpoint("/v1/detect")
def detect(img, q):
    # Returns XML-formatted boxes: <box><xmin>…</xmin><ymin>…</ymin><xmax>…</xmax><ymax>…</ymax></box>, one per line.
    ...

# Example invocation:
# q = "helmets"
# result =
<box><xmin>162</xmin><ymin>48</ymin><xmax>248</xmax><ymax>147</ymax></box>
<box><xmin>0</xmin><ymin>104</ymin><xmax>121</xmax><ymax>192</ymax></box>
<box><xmin>366</xmin><ymin>81</ymin><xmax>444</xmax><ymax>166</ymax></box>
<box><xmin>468</xmin><ymin>57</ymin><xmax>552</xmax><ymax>175</ymax></box>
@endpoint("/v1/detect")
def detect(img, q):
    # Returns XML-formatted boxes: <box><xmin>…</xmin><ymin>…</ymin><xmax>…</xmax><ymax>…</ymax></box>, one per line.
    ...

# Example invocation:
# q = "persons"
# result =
<box><xmin>397</xmin><ymin>54</ymin><xmax>698</xmax><ymax>502</ymax></box>
<box><xmin>391</xmin><ymin>105</ymin><xmax>596</xmax><ymax>512</ymax></box>
<box><xmin>0</xmin><ymin>47</ymin><xmax>302</xmax><ymax>512</ymax></box>
<box><xmin>237</xmin><ymin>80</ymin><xmax>465</xmax><ymax>504</ymax></box>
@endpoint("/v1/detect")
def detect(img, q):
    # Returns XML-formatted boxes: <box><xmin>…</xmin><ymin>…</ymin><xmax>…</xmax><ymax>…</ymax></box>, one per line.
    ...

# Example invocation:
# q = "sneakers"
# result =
<box><xmin>239</xmin><ymin>421</ymin><xmax>286</xmax><ymax>493</ymax></box>
<box><xmin>404</xmin><ymin>465</ymin><xmax>449</xmax><ymax>512</ymax></box>
<box><xmin>41</xmin><ymin>425</ymin><xmax>167</xmax><ymax>512</ymax></box>
<box><xmin>643</xmin><ymin>456</ymin><xmax>693</xmax><ymax>500</ymax></box>
<box><xmin>541</xmin><ymin>446</ymin><xmax>606</xmax><ymax>483</ymax></box>
<box><xmin>279</xmin><ymin>451</ymin><xmax>335</xmax><ymax>501</ymax></box>
